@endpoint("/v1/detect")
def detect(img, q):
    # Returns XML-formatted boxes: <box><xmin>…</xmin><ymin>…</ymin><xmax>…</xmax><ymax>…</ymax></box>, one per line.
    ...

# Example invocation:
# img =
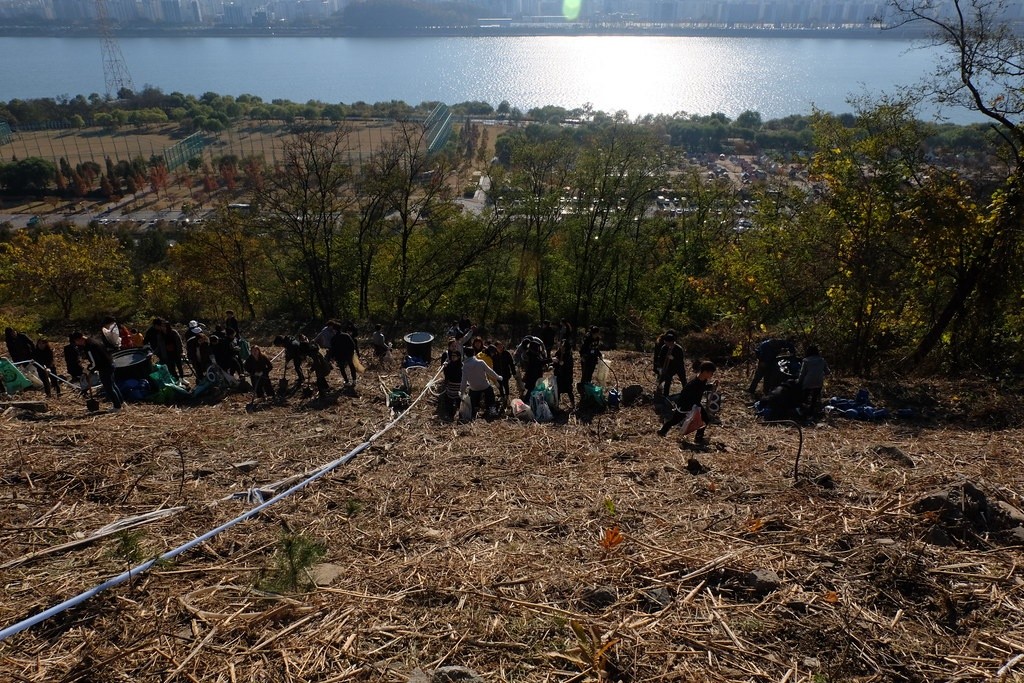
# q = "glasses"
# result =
<box><xmin>451</xmin><ymin>355</ymin><xmax>458</xmax><ymax>357</ymax></box>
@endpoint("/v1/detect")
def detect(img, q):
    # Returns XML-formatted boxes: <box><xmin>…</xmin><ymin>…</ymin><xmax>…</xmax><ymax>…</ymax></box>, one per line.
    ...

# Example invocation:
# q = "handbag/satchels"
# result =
<box><xmin>510</xmin><ymin>399</ymin><xmax>534</xmax><ymax>421</ymax></box>
<box><xmin>598</xmin><ymin>354</ymin><xmax>612</xmax><ymax>380</ymax></box>
<box><xmin>577</xmin><ymin>382</ymin><xmax>607</xmax><ymax>413</ymax></box>
<box><xmin>530</xmin><ymin>376</ymin><xmax>559</xmax><ymax>423</ymax></box>
<box><xmin>460</xmin><ymin>394</ymin><xmax>472</xmax><ymax>419</ymax></box>
<box><xmin>679</xmin><ymin>404</ymin><xmax>706</xmax><ymax>436</ymax></box>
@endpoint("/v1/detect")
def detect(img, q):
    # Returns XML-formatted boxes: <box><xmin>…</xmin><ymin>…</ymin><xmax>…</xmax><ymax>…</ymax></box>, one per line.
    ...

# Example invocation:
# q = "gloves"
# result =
<box><xmin>498</xmin><ymin>376</ymin><xmax>502</xmax><ymax>380</ymax></box>
<box><xmin>459</xmin><ymin>395</ymin><xmax>465</xmax><ymax>400</ymax></box>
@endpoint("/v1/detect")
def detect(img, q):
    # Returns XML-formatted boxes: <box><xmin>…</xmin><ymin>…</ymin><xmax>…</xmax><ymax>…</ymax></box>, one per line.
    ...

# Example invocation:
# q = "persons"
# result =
<box><xmin>743</xmin><ymin>333</ymin><xmax>830</xmax><ymax>421</ymax></box>
<box><xmin>459</xmin><ymin>314</ymin><xmax>472</xmax><ymax>346</ymax></box>
<box><xmin>657</xmin><ymin>360</ymin><xmax>716</xmax><ymax>445</ymax></box>
<box><xmin>441</xmin><ymin>325</ymin><xmax>603</xmax><ymax>421</ymax></box>
<box><xmin>6</xmin><ymin>310</ymin><xmax>275</xmax><ymax>413</ymax></box>
<box><xmin>652</xmin><ymin>329</ymin><xmax>688</xmax><ymax>396</ymax></box>
<box><xmin>373</xmin><ymin>324</ymin><xmax>389</xmax><ymax>359</ymax></box>
<box><xmin>274</xmin><ymin>320</ymin><xmax>360</xmax><ymax>396</ymax></box>
<box><xmin>102</xmin><ymin>206</ymin><xmax>111</xmax><ymax>214</ymax></box>
<box><xmin>179</xmin><ymin>202</ymin><xmax>187</xmax><ymax>215</ymax></box>
<box><xmin>560</xmin><ymin>318</ymin><xmax>572</xmax><ymax>343</ymax></box>
<box><xmin>540</xmin><ymin>321</ymin><xmax>555</xmax><ymax>356</ymax></box>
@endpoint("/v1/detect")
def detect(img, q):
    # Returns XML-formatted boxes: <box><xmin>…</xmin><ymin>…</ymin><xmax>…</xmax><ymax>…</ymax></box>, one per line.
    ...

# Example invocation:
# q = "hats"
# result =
<box><xmin>189</xmin><ymin>320</ymin><xmax>198</xmax><ymax>327</ymax></box>
<box><xmin>153</xmin><ymin>317</ymin><xmax>171</xmax><ymax>326</ymax></box>
<box><xmin>191</xmin><ymin>327</ymin><xmax>202</xmax><ymax>333</ymax></box>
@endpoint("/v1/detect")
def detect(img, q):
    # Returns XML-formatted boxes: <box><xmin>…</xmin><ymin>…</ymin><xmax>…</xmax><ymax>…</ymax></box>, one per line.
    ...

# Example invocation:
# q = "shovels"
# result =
<box><xmin>302</xmin><ymin>372</ymin><xmax>313</xmax><ymax>397</ymax></box>
<box><xmin>84</xmin><ymin>375</ymin><xmax>101</xmax><ymax>413</ymax></box>
<box><xmin>278</xmin><ymin>362</ymin><xmax>290</xmax><ymax>393</ymax></box>
<box><xmin>246</xmin><ymin>376</ymin><xmax>262</xmax><ymax>414</ymax></box>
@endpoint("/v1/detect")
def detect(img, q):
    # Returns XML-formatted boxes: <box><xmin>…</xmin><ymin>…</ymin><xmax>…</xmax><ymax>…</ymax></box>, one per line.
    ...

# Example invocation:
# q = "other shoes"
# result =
<box><xmin>747</xmin><ymin>388</ymin><xmax>755</xmax><ymax>393</ymax></box>
<box><xmin>57</xmin><ymin>393</ymin><xmax>63</xmax><ymax>398</ymax></box>
<box><xmin>352</xmin><ymin>380</ymin><xmax>357</xmax><ymax>385</ymax></box>
<box><xmin>343</xmin><ymin>381</ymin><xmax>350</xmax><ymax>385</ymax></box>
<box><xmin>109</xmin><ymin>402</ymin><xmax>129</xmax><ymax>413</ymax></box>
<box><xmin>46</xmin><ymin>393</ymin><xmax>51</xmax><ymax>398</ymax></box>
<box><xmin>694</xmin><ymin>436</ymin><xmax>708</xmax><ymax>445</ymax></box>
<box><xmin>295</xmin><ymin>379</ymin><xmax>305</xmax><ymax>382</ymax></box>
<box><xmin>258</xmin><ymin>400</ymin><xmax>265</xmax><ymax>402</ymax></box>
